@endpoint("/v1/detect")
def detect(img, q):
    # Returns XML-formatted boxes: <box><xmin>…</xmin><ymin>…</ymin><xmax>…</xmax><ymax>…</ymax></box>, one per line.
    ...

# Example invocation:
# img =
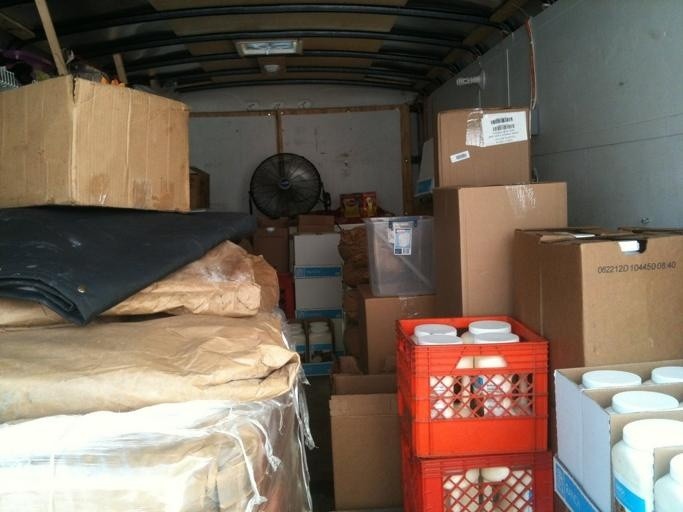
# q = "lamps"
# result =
<box><xmin>454</xmin><ymin>69</ymin><xmax>485</xmax><ymax>89</ymax></box>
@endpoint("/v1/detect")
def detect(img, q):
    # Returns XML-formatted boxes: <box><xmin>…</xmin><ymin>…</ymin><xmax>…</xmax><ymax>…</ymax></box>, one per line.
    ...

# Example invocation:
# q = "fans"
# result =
<box><xmin>249</xmin><ymin>152</ymin><xmax>329</xmax><ymax>220</ymax></box>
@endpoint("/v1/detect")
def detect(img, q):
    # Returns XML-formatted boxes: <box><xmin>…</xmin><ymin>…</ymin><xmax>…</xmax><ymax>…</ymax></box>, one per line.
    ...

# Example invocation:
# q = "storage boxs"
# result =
<box><xmin>292</xmin><ymin>268</ymin><xmax>343</xmax><ymax>311</ymax></box>
<box><xmin>252</xmin><ymin>227</ymin><xmax>288</xmax><ymax>270</ymax></box>
<box><xmin>1</xmin><ymin>74</ymin><xmax>191</xmax><ymax>213</ymax></box>
<box><xmin>190</xmin><ymin>166</ymin><xmax>209</xmax><ymax>209</ymax></box>
<box><xmin>297</xmin><ymin>214</ymin><xmax>335</xmax><ymax>233</ymax></box>
<box><xmin>293</xmin><ymin>235</ymin><xmax>344</xmax><ymax>267</ymax></box>
